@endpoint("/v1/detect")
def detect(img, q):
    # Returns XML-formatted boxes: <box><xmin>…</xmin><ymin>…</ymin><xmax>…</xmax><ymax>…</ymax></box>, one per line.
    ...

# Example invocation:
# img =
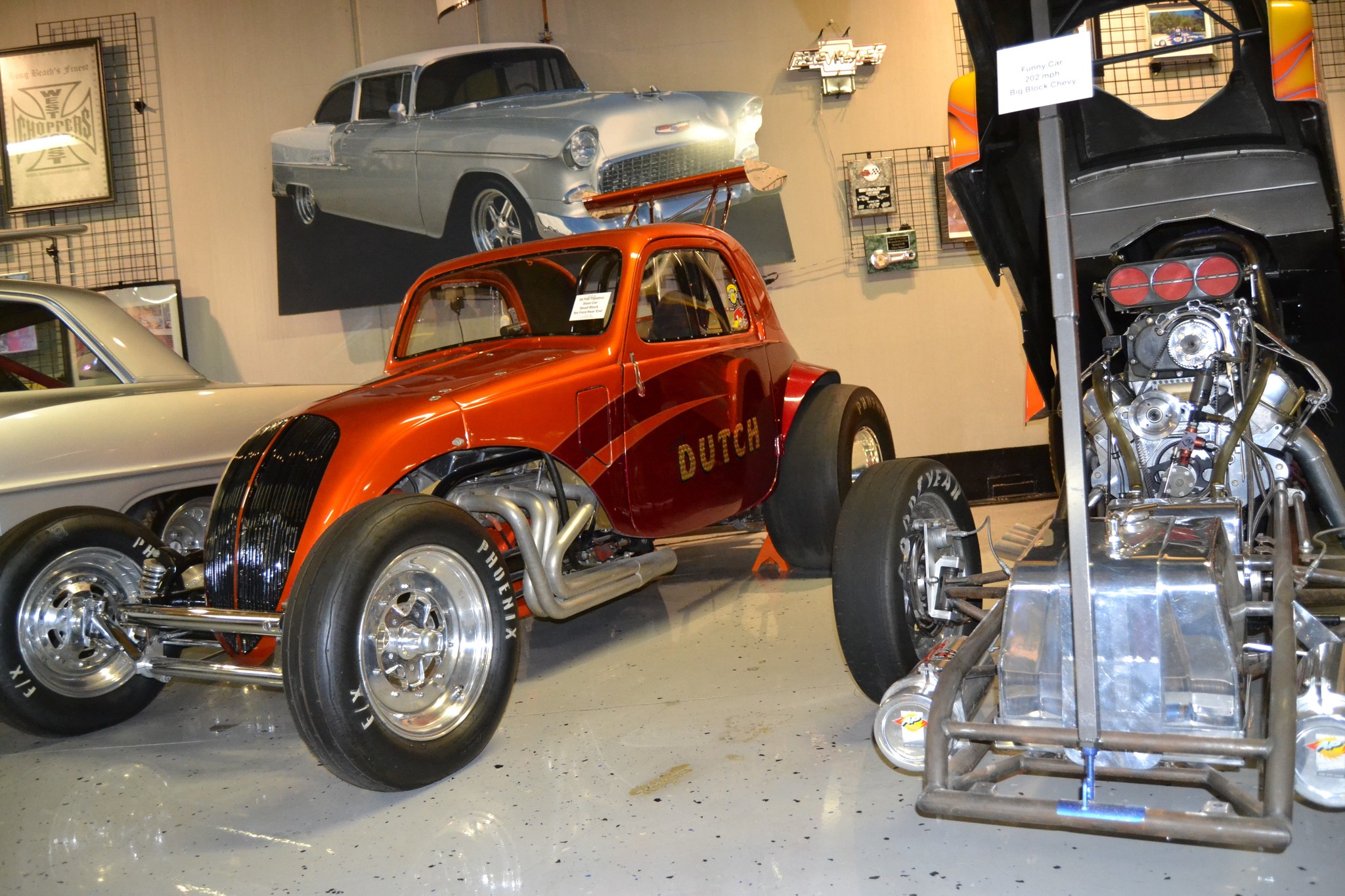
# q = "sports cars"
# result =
<box><xmin>270</xmin><ymin>39</ymin><xmax>762</xmax><ymax>261</ymax></box>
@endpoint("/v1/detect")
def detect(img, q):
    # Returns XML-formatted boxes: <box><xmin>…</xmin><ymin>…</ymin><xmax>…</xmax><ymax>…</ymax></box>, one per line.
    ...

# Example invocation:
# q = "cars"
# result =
<box><xmin>0</xmin><ymin>169</ymin><xmax>988</xmax><ymax>793</ymax></box>
<box><xmin>831</xmin><ymin>0</ymin><xmax>1345</xmax><ymax>855</ymax></box>
<box><xmin>0</xmin><ymin>281</ymin><xmax>364</xmax><ymax>562</ymax></box>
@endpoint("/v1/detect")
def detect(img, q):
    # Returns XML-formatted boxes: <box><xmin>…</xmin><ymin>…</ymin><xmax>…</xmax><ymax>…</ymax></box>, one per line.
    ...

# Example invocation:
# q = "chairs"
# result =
<box><xmin>654</xmin><ymin>291</ymin><xmax>706</xmax><ymax>341</ymax></box>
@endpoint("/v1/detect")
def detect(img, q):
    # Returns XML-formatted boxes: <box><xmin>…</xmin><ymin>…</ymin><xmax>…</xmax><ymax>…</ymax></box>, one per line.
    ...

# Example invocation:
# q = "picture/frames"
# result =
<box><xmin>0</xmin><ymin>37</ymin><xmax>117</xmax><ymax>213</ymax></box>
<box><xmin>934</xmin><ymin>156</ymin><xmax>975</xmax><ymax>244</ymax></box>
